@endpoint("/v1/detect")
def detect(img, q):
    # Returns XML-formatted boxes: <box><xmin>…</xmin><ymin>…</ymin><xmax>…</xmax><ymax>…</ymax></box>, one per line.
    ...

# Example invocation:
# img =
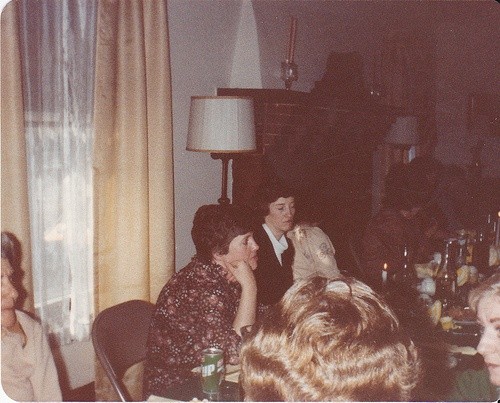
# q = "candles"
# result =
<box><xmin>382</xmin><ymin>262</ymin><xmax>388</xmax><ymax>284</ymax></box>
<box><xmin>288</xmin><ymin>19</ymin><xmax>297</xmax><ymax>64</ymax></box>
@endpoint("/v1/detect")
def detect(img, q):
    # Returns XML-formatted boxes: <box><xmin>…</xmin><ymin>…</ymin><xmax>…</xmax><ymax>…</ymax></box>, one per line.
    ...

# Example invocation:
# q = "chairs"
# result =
<box><xmin>91</xmin><ymin>298</ymin><xmax>157</xmax><ymax>403</ymax></box>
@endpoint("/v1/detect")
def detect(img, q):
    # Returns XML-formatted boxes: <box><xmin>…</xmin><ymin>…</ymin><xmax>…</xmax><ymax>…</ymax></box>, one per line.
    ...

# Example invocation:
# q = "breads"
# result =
<box><xmin>416</xmin><ymin>264</ymin><xmax>479</xmax><ymax>287</ymax></box>
<box><xmin>427</xmin><ymin>300</ymin><xmax>442</xmax><ymax>326</ymax></box>
<box><xmin>447</xmin><ymin>306</ymin><xmax>475</xmax><ymax>321</ymax></box>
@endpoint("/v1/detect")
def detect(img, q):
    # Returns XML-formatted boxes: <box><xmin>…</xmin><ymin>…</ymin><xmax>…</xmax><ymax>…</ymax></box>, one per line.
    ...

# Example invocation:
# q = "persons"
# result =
<box><xmin>1</xmin><ymin>238</ymin><xmax>63</xmax><ymax>402</ymax></box>
<box><xmin>142</xmin><ymin>205</ymin><xmax>273</xmax><ymax>402</ymax></box>
<box><xmin>238</xmin><ymin>273</ymin><xmax>416</xmax><ymax>402</ymax></box>
<box><xmin>251</xmin><ymin>180</ymin><xmax>343</xmax><ymax>307</ymax></box>
<box><xmin>467</xmin><ymin>274</ymin><xmax>500</xmax><ymax>387</ymax></box>
<box><xmin>366</xmin><ymin>174</ymin><xmax>431</xmax><ymax>319</ymax></box>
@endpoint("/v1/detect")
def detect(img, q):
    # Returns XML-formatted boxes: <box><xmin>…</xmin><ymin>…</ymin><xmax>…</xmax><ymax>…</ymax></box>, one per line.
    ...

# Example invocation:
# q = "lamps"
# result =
<box><xmin>185</xmin><ymin>96</ymin><xmax>258</xmax><ymax>205</ymax></box>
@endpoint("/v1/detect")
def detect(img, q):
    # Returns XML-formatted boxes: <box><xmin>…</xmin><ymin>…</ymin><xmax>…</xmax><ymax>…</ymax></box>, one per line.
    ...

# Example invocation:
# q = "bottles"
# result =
<box><xmin>454</xmin><ymin>231</ymin><xmax>468</xmax><ymax>294</ymax></box>
<box><xmin>436</xmin><ymin>242</ymin><xmax>455</xmax><ymax>306</ymax></box>
<box><xmin>399</xmin><ymin>243</ymin><xmax>416</xmax><ymax>291</ymax></box>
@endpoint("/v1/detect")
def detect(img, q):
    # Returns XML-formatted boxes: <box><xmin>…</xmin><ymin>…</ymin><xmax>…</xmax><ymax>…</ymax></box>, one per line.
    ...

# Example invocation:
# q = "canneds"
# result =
<box><xmin>201</xmin><ymin>348</ymin><xmax>226</xmax><ymax>395</ymax></box>
